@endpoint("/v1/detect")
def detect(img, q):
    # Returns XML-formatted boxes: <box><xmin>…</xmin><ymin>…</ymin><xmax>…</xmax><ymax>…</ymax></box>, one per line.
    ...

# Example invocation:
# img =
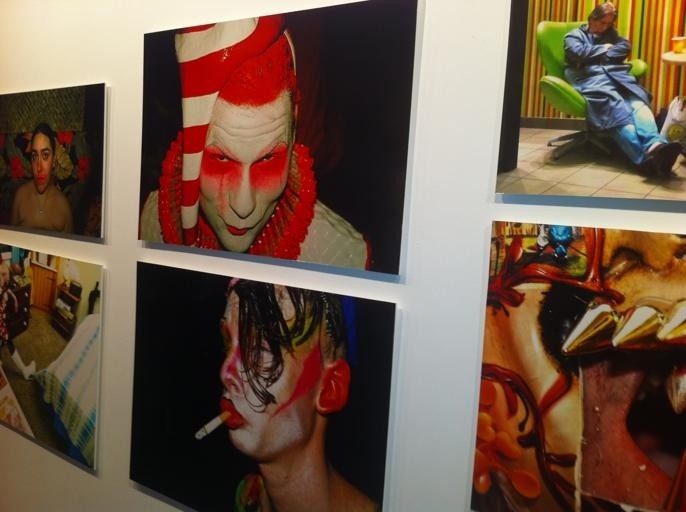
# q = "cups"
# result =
<box><xmin>672</xmin><ymin>37</ymin><xmax>684</xmax><ymax>54</ymax></box>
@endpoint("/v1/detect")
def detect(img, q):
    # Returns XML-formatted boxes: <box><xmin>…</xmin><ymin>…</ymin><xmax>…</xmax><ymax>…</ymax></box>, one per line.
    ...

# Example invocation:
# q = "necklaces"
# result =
<box><xmin>34</xmin><ymin>186</ymin><xmax>51</xmax><ymax>215</ymax></box>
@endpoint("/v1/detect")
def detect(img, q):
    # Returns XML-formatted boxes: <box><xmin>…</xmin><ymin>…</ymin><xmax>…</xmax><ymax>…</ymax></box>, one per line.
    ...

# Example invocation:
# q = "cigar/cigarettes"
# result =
<box><xmin>195</xmin><ymin>410</ymin><xmax>231</xmax><ymax>441</ymax></box>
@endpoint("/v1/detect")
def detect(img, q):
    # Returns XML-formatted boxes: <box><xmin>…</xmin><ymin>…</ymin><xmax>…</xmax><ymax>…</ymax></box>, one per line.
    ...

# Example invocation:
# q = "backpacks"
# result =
<box><xmin>4</xmin><ymin>282</ymin><xmax>31</xmax><ymax>341</ymax></box>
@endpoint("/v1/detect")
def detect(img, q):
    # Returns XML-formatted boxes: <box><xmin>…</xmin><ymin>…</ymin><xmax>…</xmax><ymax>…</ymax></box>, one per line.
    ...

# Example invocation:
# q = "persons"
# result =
<box><xmin>8</xmin><ymin>122</ymin><xmax>72</xmax><ymax>235</ymax></box>
<box><xmin>0</xmin><ymin>263</ymin><xmax>36</xmax><ymax>380</ymax></box>
<box><xmin>561</xmin><ymin>1</ymin><xmax>682</xmax><ymax>178</ymax></box>
<box><xmin>218</xmin><ymin>278</ymin><xmax>381</xmax><ymax>511</ymax></box>
<box><xmin>138</xmin><ymin>84</ymin><xmax>373</xmax><ymax>272</ymax></box>
<box><xmin>476</xmin><ymin>219</ymin><xmax>686</xmax><ymax>511</ymax></box>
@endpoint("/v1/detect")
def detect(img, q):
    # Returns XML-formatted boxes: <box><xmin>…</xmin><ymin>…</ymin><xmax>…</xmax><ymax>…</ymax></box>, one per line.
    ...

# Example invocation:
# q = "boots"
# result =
<box><xmin>11</xmin><ymin>348</ymin><xmax>36</xmax><ymax>382</ymax></box>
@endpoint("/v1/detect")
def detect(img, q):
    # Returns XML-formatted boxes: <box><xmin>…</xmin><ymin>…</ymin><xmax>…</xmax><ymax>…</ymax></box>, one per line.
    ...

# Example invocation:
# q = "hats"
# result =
<box><xmin>174</xmin><ymin>14</ymin><xmax>299</xmax><ymax>247</ymax></box>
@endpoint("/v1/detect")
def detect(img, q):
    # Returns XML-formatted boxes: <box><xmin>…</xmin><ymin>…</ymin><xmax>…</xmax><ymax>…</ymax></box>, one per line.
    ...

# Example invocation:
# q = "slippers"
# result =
<box><xmin>638</xmin><ymin>140</ymin><xmax>683</xmax><ymax>178</ymax></box>
